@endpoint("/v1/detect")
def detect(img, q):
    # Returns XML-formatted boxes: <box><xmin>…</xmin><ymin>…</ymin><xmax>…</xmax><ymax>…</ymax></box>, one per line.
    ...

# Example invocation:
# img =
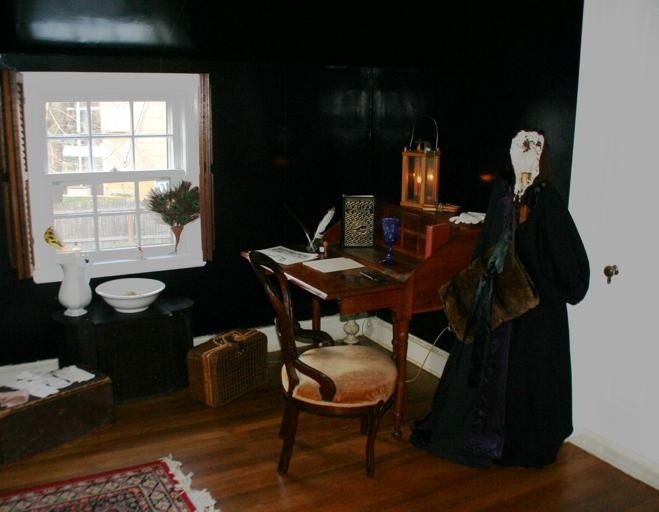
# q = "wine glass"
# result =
<box><xmin>380</xmin><ymin>218</ymin><xmax>399</xmax><ymax>266</ymax></box>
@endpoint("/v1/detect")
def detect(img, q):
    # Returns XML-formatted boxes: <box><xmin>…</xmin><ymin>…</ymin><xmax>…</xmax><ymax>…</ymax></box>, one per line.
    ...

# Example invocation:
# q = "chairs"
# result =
<box><xmin>248</xmin><ymin>250</ymin><xmax>399</xmax><ymax>475</ymax></box>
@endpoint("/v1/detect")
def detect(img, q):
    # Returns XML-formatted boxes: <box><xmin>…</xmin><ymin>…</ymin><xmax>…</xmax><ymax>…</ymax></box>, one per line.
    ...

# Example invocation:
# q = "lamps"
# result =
<box><xmin>399</xmin><ymin>114</ymin><xmax>443</xmax><ymax>210</ymax></box>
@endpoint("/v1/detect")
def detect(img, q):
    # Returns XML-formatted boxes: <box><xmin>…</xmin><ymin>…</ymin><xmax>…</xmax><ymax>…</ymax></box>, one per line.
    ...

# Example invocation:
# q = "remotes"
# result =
<box><xmin>360</xmin><ymin>270</ymin><xmax>385</xmax><ymax>283</ymax></box>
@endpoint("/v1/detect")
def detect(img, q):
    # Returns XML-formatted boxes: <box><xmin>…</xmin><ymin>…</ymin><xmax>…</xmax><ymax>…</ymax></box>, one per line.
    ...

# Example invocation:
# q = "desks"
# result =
<box><xmin>1</xmin><ymin>360</ymin><xmax>116</xmax><ymax>464</ymax></box>
<box><xmin>42</xmin><ymin>297</ymin><xmax>195</xmax><ymax>400</ymax></box>
<box><xmin>239</xmin><ymin>203</ymin><xmax>483</xmax><ymax>441</ymax></box>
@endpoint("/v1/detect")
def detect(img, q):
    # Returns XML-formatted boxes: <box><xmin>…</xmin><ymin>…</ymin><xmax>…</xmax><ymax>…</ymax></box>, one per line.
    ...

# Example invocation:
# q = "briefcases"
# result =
<box><xmin>187</xmin><ymin>327</ymin><xmax>267</xmax><ymax>409</ymax></box>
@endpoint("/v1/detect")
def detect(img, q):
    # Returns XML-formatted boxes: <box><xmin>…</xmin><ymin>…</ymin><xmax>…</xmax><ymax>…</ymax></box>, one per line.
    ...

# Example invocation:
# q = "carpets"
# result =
<box><xmin>1</xmin><ymin>454</ymin><xmax>219</xmax><ymax>512</ymax></box>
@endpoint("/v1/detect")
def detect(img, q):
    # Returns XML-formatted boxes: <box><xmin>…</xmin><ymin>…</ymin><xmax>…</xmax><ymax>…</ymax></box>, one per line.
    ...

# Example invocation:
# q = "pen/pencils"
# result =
<box><xmin>304</xmin><ymin>231</ymin><xmax>315</xmax><ymax>253</ymax></box>
<box><xmin>307</xmin><ymin>206</ymin><xmax>336</xmax><ymax>252</ymax></box>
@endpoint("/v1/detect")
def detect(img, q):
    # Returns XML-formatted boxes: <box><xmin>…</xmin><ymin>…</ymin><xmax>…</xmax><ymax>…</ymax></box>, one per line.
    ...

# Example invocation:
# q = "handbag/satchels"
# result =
<box><xmin>437</xmin><ymin>194</ymin><xmax>538</xmax><ymax>346</ymax></box>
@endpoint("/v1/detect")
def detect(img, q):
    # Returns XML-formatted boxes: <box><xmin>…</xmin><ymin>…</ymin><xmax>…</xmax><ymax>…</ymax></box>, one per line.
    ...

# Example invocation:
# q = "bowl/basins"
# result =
<box><xmin>95</xmin><ymin>278</ymin><xmax>166</xmax><ymax>313</ymax></box>
